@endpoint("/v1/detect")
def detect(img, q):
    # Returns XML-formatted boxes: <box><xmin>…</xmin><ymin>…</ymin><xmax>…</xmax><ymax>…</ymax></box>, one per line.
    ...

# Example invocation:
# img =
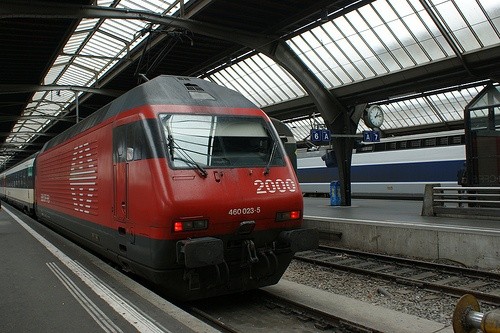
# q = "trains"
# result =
<box><xmin>0</xmin><ymin>75</ymin><xmax>321</xmax><ymax>310</ymax></box>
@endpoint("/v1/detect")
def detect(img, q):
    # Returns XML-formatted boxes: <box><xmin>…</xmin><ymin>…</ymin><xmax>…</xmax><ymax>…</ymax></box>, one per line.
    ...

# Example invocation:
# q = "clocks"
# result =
<box><xmin>364</xmin><ymin>105</ymin><xmax>384</xmax><ymax>128</ymax></box>
